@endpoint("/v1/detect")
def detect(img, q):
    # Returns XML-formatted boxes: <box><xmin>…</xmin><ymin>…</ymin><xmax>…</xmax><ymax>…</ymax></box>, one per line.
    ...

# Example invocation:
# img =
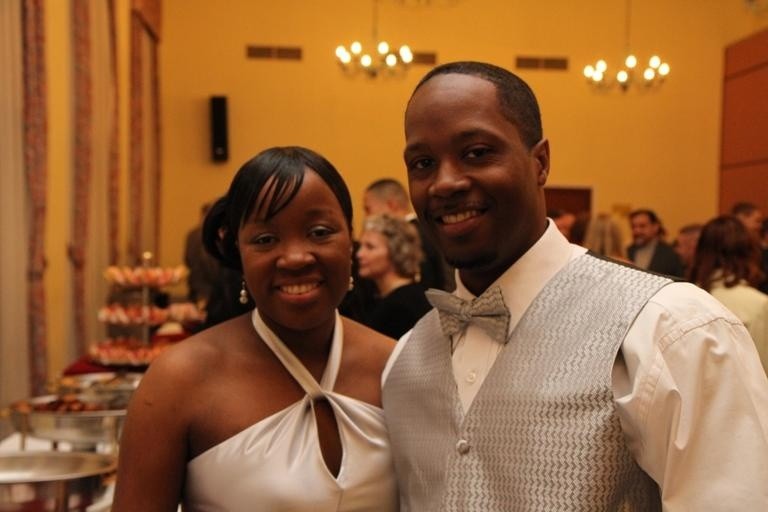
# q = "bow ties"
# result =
<box><xmin>424</xmin><ymin>284</ymin><xmax>511</xmax><ymax>347</ymax></box>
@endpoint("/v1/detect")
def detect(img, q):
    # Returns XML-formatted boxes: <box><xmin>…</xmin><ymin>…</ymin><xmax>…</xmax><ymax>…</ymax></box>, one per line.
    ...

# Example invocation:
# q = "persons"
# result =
<box><xmin>380</xmin><ymin>61</ymin><xmax>768</xmax><ymax>512</ymax></box>
<box><xmin>109</xmin><ymin>141</ymin><xmax>402</xmax><ymax>512</ymax></box>
<box><xmin>337</xmin><ymin>178</ymin><xmax>456</xmax><ymax>342</ymax></box>
<box><xmin>550</xmin><ymin>200</ymin><xmax>768</xmax><ymax>378</ymax></box>
<box><xmin>185</xmin><ymin>202</ymin><xmax>256</xmax><ymax>331</ymax></box>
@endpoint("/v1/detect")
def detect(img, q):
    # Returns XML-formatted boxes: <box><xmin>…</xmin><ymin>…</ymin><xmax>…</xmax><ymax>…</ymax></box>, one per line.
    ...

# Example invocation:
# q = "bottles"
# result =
<box><xmin>94</xmin><ymin>416</ymin><xmax>120</xmax><ymax>465</ymax></box>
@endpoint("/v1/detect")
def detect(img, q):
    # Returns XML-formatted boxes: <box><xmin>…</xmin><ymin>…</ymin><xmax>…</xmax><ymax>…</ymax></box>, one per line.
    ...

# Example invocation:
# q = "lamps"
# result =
<box><xmin>583</xmin><ymin>0</ymin><xmax>670</xmax><ymax>92</ymax></box>
<box><xmin>335</xmin><ymin>0</ymin><xmax>414</xmax><ymax>81</ymax></box>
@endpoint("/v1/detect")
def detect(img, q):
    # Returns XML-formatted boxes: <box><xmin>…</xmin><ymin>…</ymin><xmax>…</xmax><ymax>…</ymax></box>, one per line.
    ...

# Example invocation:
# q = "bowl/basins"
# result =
<box><xmin>6</xmin><ymin>391</ymin><xmax>129</xmax><ymax>447</ymax></box>
<box><xmin>0</xmin><ymin>452</ymin><xmax>121</xmax><ymax>512</ymax></box>
<box><xmin>40</xmin><ymin>372</ymin><xmax>145</xmax><ymax>395</ymax></box>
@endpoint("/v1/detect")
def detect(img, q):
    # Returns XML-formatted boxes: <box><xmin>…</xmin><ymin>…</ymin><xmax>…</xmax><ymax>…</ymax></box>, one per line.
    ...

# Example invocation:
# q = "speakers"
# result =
<box><xmin>210</xmin><ymin>96</ymin><xmax>228</xmax><ymax>163</ymax></box>
